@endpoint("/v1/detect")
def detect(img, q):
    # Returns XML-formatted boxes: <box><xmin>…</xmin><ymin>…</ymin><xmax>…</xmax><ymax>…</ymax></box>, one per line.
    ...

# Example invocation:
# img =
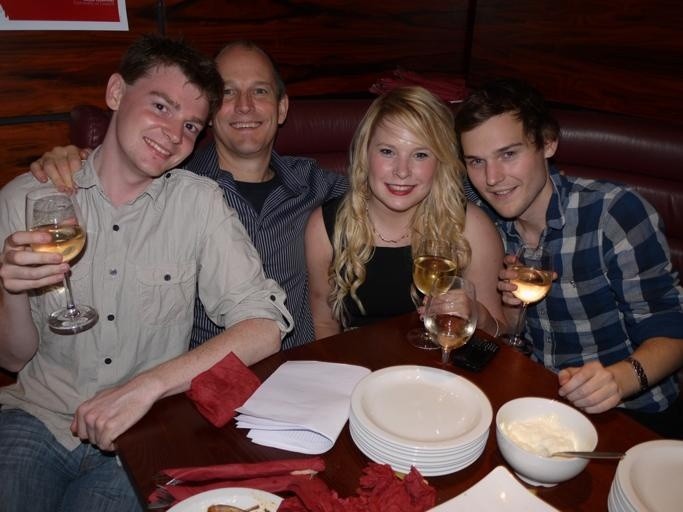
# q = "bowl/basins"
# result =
<box><xmin>495</xmin><ymin>395</ymin><xmax>599</xmax><ymax>489</ymax></box>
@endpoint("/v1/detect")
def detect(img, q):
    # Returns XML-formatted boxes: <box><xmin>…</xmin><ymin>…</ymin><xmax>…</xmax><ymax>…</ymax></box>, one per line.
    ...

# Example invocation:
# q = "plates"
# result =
<box><xmin>423</xmin><ymin>465</ymin><xmax>560</xmax><ymax>512</ymax></box>
<box><xmin>165</xmin><ymin>487</ymin><xmax>298</xmax><ymax>512</ymax></box>
<box><xmin>606</xmin><ymin>439</ymin><xmax>682</xmax><ymax>511</ymax></box>
<box><xmin>347</xmin><ymin>364</ymin><xmax>493</xmax><ymax>478</ymax></box>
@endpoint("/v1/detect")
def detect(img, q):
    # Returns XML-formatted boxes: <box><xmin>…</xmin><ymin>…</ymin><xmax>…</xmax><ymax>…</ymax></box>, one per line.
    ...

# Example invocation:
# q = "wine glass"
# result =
<box><xmin>25</xmin><ymin>185</ymin><xmax>99</xmax><ymax>335</ymax></box>
<box><xmin>411</xmin><ymin>235</ymin><xmax>553</xmax><ymax>369</ymax></box>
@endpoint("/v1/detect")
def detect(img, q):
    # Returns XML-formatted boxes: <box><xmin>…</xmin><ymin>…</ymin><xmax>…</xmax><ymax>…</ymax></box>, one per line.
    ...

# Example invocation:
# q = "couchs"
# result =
<box><xmin>70</xmin><ymin>71</ymin><xmax>682</xmax><ymax>287</ymax></box>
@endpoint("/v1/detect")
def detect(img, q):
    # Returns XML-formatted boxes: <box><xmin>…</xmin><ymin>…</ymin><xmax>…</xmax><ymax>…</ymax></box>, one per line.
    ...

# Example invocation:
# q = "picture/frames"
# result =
<box><xmin>0</xmin><ymin>0</ymin><xmax>130</xmax><ymax>31</ymax></box>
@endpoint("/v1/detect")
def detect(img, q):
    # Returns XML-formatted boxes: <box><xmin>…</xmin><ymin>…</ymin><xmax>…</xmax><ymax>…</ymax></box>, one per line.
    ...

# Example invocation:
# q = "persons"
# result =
<box><xmin>29</xmin><ymin>43</ymin><xmax>480</xmax><ymax>348</ymax></box>
<box><xmin>304</xmin><ymin>77</ymin><xmax>683</xmax><ymax>436</ymax></box>
<box><xmin>0</xmin><ymin>37</ymin><xmax>296</xmax><ymax>512</ymax></box>
